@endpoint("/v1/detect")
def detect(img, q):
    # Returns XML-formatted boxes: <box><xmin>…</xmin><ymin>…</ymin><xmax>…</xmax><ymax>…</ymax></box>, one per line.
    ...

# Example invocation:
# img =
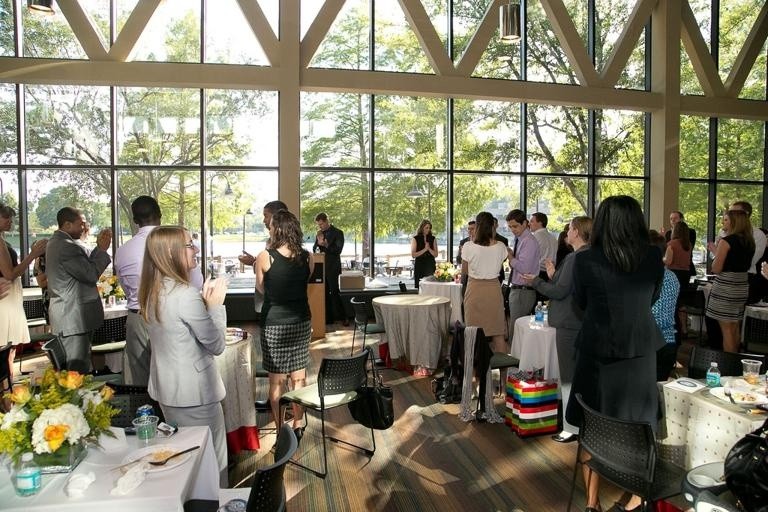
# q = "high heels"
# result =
<box><xmin>294</xmin><ymin>428</ymin><xmax>303</xmax><ymax>448</ymax></box>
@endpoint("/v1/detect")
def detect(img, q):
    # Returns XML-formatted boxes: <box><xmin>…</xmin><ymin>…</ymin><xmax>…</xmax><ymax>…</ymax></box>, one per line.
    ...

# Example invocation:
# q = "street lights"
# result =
<box><xmin>409</xmin><ymin>175</ymin><xmax>434</xmax><ymax>237</ymax></box>
<box><xmin>209</xmin><ymin>173</ymin><xmax>233</xmax><ymax>280</ymax></box>
<box><xmin>240</xmin><ymin>209</ymin><xmax>253</xmax><ymax>274</ymax></box>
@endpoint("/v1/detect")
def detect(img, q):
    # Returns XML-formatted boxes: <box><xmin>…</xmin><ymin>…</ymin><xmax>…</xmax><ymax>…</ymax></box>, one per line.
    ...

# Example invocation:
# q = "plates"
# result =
<box><xmin>709</xmin><ymin>387</ymin><xmax>768</xmax><ymax>406</ymax></box>
<box><xmin>124</xmin><ymin>443</ymin><xmax>194</xmax><ymax>472</ymax></box>
<box><xmin>224</xmin><ymin>328</ymin><xmax>247</xmax><ymax>344</ymax></box>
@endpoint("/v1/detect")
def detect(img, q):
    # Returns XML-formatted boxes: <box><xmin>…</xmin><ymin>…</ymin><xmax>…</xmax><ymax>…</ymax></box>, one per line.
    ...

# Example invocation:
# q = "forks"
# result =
<box><xmin>150</xmin><ymin>445</ymin><xmax>200</xmax><ymax>466</ymax></box>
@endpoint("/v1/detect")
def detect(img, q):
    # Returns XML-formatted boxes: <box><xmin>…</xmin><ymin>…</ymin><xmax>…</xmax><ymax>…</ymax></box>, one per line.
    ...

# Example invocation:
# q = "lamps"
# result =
<box><xmin>499</xmin><ymin>0</ymin><xmax>521</xmax><ymax>43</ymax></box>
<box><xmin>26</xmin><ymin>0</ymin><xmax>56</xmax><ymax>16</ymax></box>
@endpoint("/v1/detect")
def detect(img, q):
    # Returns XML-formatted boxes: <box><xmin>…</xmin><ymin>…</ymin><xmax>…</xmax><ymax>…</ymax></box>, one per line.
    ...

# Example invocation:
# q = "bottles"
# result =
<box><xmin>316</xmin><ymin>247</ymin><xmax>321</xmax><ymax>254</ymax></box>
<box><xmin>529</xmin><ymin>301</ymin><xmax>549</xmax><ymax>330</ymax></box>
<box><xmin>766</xmin><ymin>370</ymin><xmax>768</xmax><ymax>383</ymax></box>
<box><xmin>14</xmin><ymin>452</ymin><xmax>42</xmax><ymax>496</ymax></box>
<box><xmin>706</xmin><ymin>362</ymin><xmax>720</xmax><ymax>387</ymax></box>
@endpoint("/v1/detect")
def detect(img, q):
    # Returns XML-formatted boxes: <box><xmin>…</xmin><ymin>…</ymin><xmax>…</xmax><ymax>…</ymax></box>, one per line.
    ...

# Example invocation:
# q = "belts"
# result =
<box><xmin>131</xmin><ymin>308</ymin><xmax>142</xmax><ymax>316</ymax></box>
<box><xmin>510</xmin><ymin>286</ymin><xmax>532</xmax><ymax>291</ymax></box>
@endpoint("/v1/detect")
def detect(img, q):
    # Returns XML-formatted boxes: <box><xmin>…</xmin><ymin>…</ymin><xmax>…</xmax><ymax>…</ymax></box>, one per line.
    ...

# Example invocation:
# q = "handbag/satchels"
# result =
<box><xmin>505</xmin><ymin>373</ymin><xmax>558</xmax><ymax>439</ymax></box>
<box><xmin>724</xmin><ymin>419</ymin><xmax>767</xmax><ymax>510</ymax></box>
<box><xmin>432</xmin><ymin>376</ymin><xmax>461</xmax><ymax>405</ymax></box>
<box><xmin>348</xmin><ymin>386</ymin><xmax>394</xmax><ymax>430</ymax></box>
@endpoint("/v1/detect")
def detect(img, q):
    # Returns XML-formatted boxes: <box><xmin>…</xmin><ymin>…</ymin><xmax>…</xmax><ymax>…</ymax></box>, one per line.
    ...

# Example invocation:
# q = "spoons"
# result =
<box><xmin>723</xmin><ymin>388</ymin><xmax>737</xmax><ymax>404</ymax></box>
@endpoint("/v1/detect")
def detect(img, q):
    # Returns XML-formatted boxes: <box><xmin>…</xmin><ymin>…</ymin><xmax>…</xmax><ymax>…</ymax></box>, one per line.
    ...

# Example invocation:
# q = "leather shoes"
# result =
<box><xmin>552</xmin><ymin>433</ymin><xmax>576</xmax><ymax>443</ymax></box>
<box><xmin>616</xmin><ymin>502</ymin><xmax>647</xmax><ymax>511</ymax></box>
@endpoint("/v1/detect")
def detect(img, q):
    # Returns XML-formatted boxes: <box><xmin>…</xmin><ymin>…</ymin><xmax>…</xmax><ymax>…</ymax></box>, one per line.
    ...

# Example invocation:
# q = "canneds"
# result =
<box><xmin>135</xmin><ymin>405</ymin><xmax>156</xmax><ymax>439</ymax></box>
<box><xmin>108</xmin><ymin>295</ymin><xmax>116</xmax><ymax>306</ymax></box>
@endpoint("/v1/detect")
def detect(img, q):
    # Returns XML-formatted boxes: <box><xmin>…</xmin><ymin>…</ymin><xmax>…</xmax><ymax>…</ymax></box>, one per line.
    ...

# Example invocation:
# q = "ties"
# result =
<box><xmin>508</xmin><ymin>239</ymin><xmax>518</xmax><ymax>285</ymax></box>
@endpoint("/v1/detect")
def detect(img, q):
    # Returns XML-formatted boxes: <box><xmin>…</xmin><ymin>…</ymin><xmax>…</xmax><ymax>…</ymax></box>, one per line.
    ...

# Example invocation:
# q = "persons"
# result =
<box><xmin>1</xmin><ymin>204</ymin><xmax>49</xmax><ymax>415</ymax></box>
<box><xmin>137</xmin><ymin>223</ymin><xmax>232</xmax><ymax>489</ymax></box>
<box><xmin>411</xmin><ymin>219</ymin><xmax>438</xmax><ymax>288</ymax></box>
<box><xmin>570</xmin><ymin>196</ymin><xmax>664</xmax><ymax>512</ymax></box>
<box><xmin>312</xmin><ymin>213</ymin><xmax>350</xmax><ymax>326</ymax></box>
<box><xmin>705</xmin><ymin>200</ymin><xmax>766</xmax><ymax>354</ymax></box>
<box><xmin>362</xmin><ymin>254</ymin><xmax>369</xmax><ymax>268</ymax></box>
<box><xmin>455</xmin><ymin>209</ymin><xmax>697</xmax><ymax>441</ymax></box>
<box><xmin>254</xmin><ymin>211</ymin><xmax>310</xmax><ymax>448</ymax></box>
<box><xmin>31</xmin><ymin>207</ymin><xmax>115</xmax><ymax>378</ymax></box>
<box><xmin>115</xmin><ymin>194</ymin><xmax>205</xmax><ymax>388</ymax></box>
<box><xmin>237</xmin><ymin>200</ymin><xmax>296</xmax><ymax>412</ymax></box>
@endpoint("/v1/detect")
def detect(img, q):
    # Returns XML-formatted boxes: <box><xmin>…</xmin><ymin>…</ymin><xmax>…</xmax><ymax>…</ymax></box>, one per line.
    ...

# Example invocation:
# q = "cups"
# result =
<box><xmin>740</xmin><ymin>359</ymin><xmax>764</xmax><ymax>384</ymax></box>
<box><xmin>456</xmin><ymin>264</ymin><xmax>462</xmax><ymax>284</ymax></box>
<box><xmin>131</xmin><ymin>415</ymin><xmax>162</xmax><ymax>446</ymax></box>
